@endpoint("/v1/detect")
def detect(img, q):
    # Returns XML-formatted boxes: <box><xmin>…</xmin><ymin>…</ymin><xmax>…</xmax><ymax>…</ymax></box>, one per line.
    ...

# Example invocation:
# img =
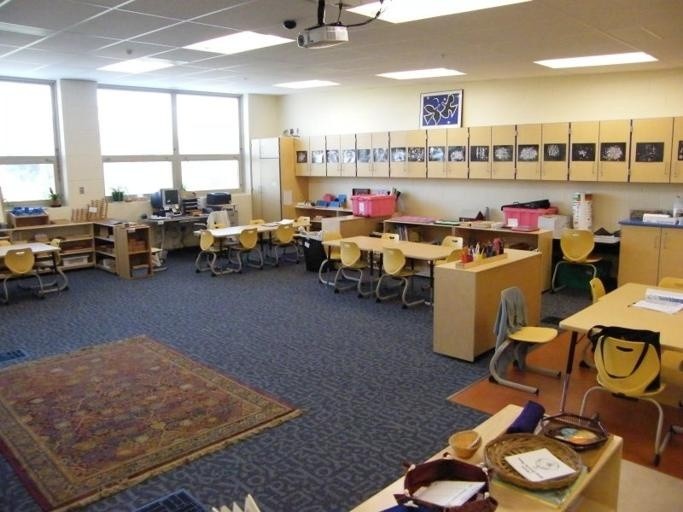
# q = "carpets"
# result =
<box><xmin>0</xmin><ymin>331</ymin><xmax>305</xmax><ymax>512</ymax></box>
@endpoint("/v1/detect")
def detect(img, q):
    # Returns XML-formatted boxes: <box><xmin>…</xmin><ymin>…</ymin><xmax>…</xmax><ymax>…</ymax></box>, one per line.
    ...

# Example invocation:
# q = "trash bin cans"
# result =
<box><xmin>303</xmin><ymin>235</ymin><xmax>327</xmax><ymax>273</ymax></box>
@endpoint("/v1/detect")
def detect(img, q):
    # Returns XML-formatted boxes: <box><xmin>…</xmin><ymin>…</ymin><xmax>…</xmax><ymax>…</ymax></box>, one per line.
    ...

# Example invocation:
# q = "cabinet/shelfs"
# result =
<box><xmin>249</xmin><ymin>137</ymin><xmax>309</xmax><ymax>221</ymax></box>
<box><xmin>294</xmin><ymin>115</ymin><xmax>683</xmax><ymax>184</ymax></box>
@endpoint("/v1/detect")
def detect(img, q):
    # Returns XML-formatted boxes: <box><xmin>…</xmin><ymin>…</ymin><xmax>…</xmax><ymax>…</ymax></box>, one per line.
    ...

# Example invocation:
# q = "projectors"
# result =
<box><xmin>298</xmin><ymin>24</ymin><xmax>349</xmax><ymax>50</ymax></box>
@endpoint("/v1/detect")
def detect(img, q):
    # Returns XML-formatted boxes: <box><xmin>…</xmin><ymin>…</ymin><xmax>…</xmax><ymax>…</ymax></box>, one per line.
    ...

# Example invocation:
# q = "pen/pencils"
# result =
<box><xmin>464</xmin><ymin>241</ymin><xmax>492</xmax><ymax>253</ymax></box>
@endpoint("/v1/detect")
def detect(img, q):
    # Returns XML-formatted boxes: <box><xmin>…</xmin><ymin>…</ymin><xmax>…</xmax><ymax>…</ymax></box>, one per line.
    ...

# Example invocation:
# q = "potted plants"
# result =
<box><xmin>47</xmin><ymin>187</ymin><xmax>64</xmax><ymax>207</ymax></box>
<box><xmin>110</xmin><ymin>187</ymin><xmax>125</xmax><ymax>202</ymax></box>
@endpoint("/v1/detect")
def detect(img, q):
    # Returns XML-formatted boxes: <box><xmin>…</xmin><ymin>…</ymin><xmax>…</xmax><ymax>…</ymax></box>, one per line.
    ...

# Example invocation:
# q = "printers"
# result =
<box><xmin>207</xmin><ymin>192</ymin><xmax>231</xmax><ymax>204</ymax></box>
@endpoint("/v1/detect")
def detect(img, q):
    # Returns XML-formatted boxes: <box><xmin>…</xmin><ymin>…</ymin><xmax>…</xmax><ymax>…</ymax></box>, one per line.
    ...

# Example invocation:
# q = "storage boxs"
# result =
<box><xmin>350</xmin><ymin>191</ymin><xmax>399</xmax><ymax>220</ymax></box>
<box><xmin>501</xmin><ymin>202</ymin><xmax>559</xmax><ymax>230</ymax></box>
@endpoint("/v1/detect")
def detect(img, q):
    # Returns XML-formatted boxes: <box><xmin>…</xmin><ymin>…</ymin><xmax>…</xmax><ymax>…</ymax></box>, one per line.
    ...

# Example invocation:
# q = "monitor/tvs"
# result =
<box><xmin>151</xmin><ymin>188</ymin><xmax>181</xmax><ymax>210</ymax></box>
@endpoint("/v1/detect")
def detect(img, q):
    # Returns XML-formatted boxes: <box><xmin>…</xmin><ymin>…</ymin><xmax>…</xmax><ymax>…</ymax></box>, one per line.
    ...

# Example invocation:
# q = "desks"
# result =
<box><xmin>433</xmin><ymin>246</ymin><xmax>543</xmax><ymax>362</ymax></box>
<box><xmin>347</xmin><ymin>405</ymin><xmax>626</xmax><ymax>512</ymax></box>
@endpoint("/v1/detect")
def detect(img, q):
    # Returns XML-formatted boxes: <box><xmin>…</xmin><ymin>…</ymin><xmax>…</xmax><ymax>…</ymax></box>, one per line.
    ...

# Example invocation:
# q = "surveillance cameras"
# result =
<box><xmin>282</xmin><ymin>19</ymin><xmax>296</xmax><ymax>30</ymax></box>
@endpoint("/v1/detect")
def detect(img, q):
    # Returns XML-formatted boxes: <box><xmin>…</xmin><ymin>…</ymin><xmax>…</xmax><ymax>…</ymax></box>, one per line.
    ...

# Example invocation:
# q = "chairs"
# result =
<box><xmin>490</xmin><ymin>286</ymin><xmax>561</xmax><ymax>393</ymax></box>
<box><xmin>552</xmin><ymin>205</ymin><xmax>683</xmax><ymax>413</ymax></box>
<box><xmin>296</xmin><ymin>202</ymin><xmax>552</xmax><ymax>308</ymax></box>
<box><xmin>577</xmin><ymin>325</ymin><xmax>672</xmax><ymax>466</ymax></box>
<box><xmin>0</xmin><ymin>207</ymin><xmax>308</xmax><ymax>301</ymax></box>
